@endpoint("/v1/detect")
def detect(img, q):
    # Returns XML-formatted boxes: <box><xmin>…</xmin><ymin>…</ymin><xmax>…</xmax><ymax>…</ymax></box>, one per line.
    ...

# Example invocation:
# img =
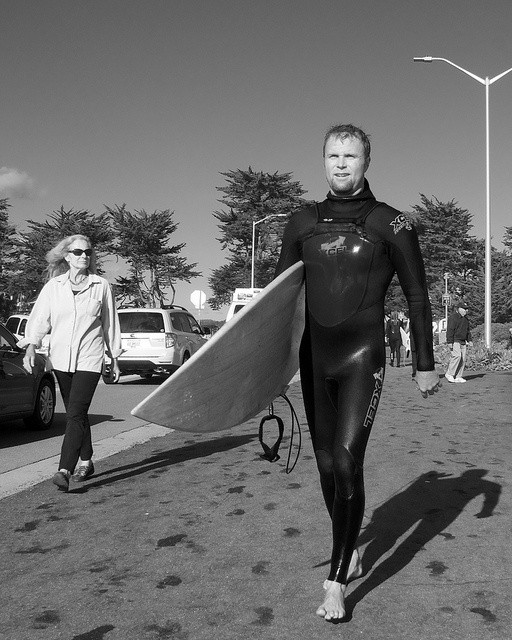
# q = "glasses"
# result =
<box><xmin>67</xmin><ymin>249</ymin><xmax>93</xmax><ymax>257</ymax></box>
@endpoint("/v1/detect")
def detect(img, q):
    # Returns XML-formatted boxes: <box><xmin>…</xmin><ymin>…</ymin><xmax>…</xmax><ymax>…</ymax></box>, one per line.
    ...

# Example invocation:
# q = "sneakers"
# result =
<box><xmin>454</xmin><ymin>378</ymin><xmax>466</xmax><ymax>383</ymax></box>
<box><xmin>388</xmin><ymin>362</ymin><xmax>394</xmax><ymax>367</ymax></box>
<box><xmin>445</xmin><ymin>372</ymin><xmax>455</xmax><ymax>383</ymax></box>
<box><xmin>73</xmin><ymin>458</ymin><xmax>96</xmax><ymax>481</ymax></box>
<box><xmin>53</xmin><ymin>472</ymin><xmax>70</xmax><ymax>491</ymax></box>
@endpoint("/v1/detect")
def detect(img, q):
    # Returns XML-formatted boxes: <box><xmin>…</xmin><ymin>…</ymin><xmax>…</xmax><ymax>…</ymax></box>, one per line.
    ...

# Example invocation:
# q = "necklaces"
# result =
<box><xmin>68</xmin><ymin>275</ymin><xmax>88</xmax><ymax>285</ymax></box>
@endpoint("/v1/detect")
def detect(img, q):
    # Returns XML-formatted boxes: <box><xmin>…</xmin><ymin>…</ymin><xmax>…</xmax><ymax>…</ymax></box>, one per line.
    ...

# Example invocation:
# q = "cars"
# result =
<box><xmin>5</xmin><ymin>315</ymin><xmax>51</xmax><ymax>354</ymax></box>
<box><xmin>0</xmin><ymin>323</ymin><xmax>56</xmax><ymax>430</ymax></box>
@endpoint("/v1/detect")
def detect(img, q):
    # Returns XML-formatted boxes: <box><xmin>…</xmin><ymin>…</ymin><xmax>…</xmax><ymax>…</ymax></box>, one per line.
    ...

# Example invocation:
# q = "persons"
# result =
<box><xmin>445</xmin><ymin>303</ymin><xmax>473</xmax><ymax>382</ymax></box>
<box><xmin>274</xmin><ymin>125</ymin><xmax>442</xmax><ymax>621</ymax></box>
<box><xmin>405</xmin><ymin>316</ymin><xmax>413</xmax><ymax>359</ymax></box>
<box><xmin>20</xmin><ymin>235</ymin><xmax>125</xmax><ymax>492</ymax></box>
<box><xmin>384</xmin><ymin>310</ymin><xmax>403</xmax><ymax>367</ymax></box>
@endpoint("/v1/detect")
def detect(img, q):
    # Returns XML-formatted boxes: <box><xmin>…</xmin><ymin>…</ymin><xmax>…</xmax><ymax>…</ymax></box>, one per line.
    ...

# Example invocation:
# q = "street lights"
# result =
<box><xmin>251</xmin><ymin>214</ymin><xmax>287</xmax><ymax>288</ymax></box>
<box><xmin>414</xmin><ymin>56</ymin><xmax>512</xmax><ymax>347</ymax></box>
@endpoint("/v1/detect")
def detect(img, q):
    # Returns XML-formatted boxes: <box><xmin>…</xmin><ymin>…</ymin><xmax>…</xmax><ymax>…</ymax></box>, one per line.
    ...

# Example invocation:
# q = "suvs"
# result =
<box><xmin>102</xmin><ymin>305</ymin><xmax>210</xmax><ymax>384</ymax></box>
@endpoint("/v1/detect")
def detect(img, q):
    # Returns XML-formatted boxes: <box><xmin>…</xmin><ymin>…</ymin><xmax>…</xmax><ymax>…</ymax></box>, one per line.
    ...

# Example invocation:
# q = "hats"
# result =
<box><xmin>457</xmin><ymin>302</ymin><xmax>470</xmax><ymax>310</ymax></box>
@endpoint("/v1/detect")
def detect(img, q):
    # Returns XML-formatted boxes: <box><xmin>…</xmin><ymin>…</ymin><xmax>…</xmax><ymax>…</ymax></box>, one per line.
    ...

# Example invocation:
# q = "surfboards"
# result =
<box><xmin>131</xmin><ymin>259</ymin><xmax>305</xmax><ymax>432</ymax></box>
<box><xmin>400</xmin><ymin>327</ymin><xmax>411</xmax><ymax>351</ymax></box>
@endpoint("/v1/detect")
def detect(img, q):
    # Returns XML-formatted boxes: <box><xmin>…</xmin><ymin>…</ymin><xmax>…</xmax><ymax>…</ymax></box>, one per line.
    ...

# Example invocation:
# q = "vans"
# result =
<box><xmin>226</xmin><ymin>288</ymin><xmax>263</xmax><ymax>322</ymax></box>
